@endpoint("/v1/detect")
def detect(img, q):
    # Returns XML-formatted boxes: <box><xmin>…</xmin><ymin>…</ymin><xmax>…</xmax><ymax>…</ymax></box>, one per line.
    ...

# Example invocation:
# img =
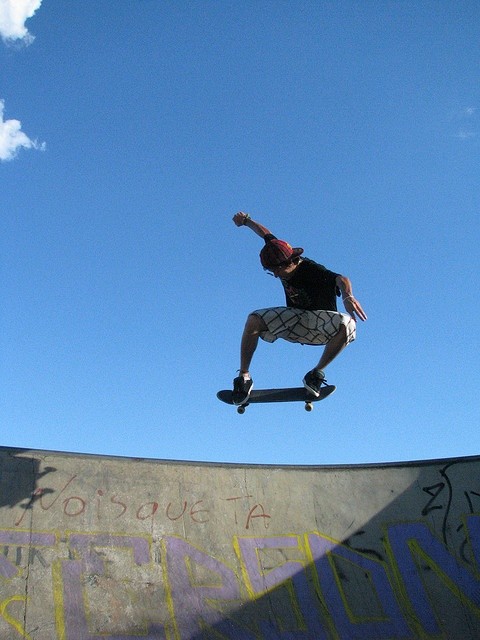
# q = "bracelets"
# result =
<box><xmin>343</xmin><ymin>295</ymin><xmax>354</xmax><ymax>300</ymax></box>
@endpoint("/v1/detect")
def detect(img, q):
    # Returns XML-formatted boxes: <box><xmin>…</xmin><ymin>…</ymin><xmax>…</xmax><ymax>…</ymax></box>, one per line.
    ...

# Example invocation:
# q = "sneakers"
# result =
<box><xmin>302</xmin><ymin>372</ymin><xmax>329</xmax><ymax>397</ymax></box>
<box><xmin>232</xmin><ymin>376</ymin><xmax>253</xmax><ymax>405</ymax></box>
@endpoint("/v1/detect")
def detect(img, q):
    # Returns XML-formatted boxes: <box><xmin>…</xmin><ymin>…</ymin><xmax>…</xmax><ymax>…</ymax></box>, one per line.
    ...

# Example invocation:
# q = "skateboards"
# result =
<box><xmin>216</xmin><ymin>384</ymin><xmax>336</xmax><ymax>414</ymax></box>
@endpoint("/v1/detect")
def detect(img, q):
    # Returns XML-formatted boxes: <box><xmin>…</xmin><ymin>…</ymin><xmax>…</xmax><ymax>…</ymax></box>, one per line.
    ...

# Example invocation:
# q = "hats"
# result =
<box><xmin>260</xmin><ymin>239</ymin><xmax>304</xmax><ymax>267</ymax></box>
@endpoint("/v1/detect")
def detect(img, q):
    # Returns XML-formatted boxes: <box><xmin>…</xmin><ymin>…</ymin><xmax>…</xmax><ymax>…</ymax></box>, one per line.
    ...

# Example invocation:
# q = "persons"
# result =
<box><xmin>231</xmin><ymin>213</ymin><xmax>368</xmax><ymax>406</ymax></box>
